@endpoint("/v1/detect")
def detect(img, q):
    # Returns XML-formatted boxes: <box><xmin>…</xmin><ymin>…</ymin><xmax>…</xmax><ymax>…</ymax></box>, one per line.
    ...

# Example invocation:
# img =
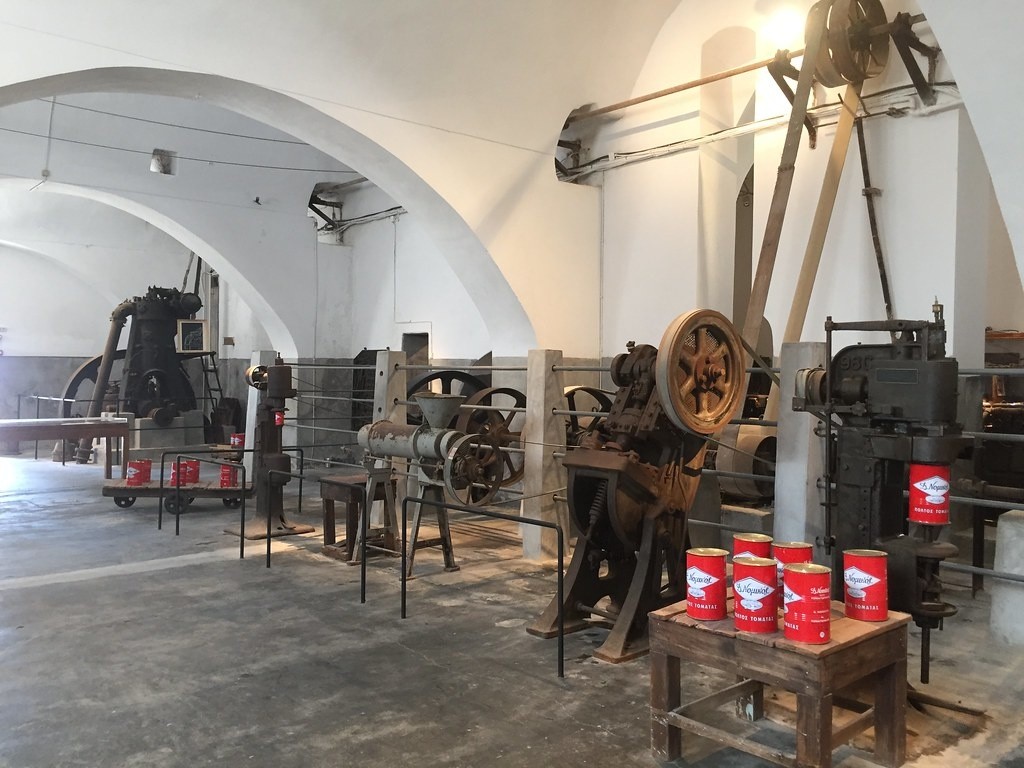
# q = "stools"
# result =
<box><xmin>321</xmin><ymin>476</ymin><xmax>398</xmax><ymax>561</ymax></box>
<box><xmin>647</xmin><ymin>587</ymin><xmax>912</xmax><ymax>768</ymax></box>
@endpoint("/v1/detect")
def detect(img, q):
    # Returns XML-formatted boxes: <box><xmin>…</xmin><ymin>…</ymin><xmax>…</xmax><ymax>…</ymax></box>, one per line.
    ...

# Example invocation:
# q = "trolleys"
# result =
<box><xmin>101</xmin><ymin>425</ymin><xmax>259</xmax><ymax>514</ymax></box>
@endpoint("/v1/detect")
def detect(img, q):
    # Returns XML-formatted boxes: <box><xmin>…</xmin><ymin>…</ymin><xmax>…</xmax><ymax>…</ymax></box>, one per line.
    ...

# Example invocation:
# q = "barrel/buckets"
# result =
<box><xmin>771</xmin><ymin>541</ymin><xmax>814</xmax><ymax>610</ymax></box>
<box><xmin>732</xmin><ymin>533</ymin><xmax>774</xmax><ymax>560</ymax></box>
<box><xmin>733</xmin><ymin>556</ymin><xmax>779</xmax><ymax>634</ymax></box>
<box><xmin>908</xmin><ymin>463</ymin><xmax>950</xmax><ymax>524</ymax></box>
<box><xmin>220</xmin><ymin>464</ymin><xmax>237</xmax><ymax>487</ymax></box>
<box><xmin>126</xmin><ymin>458</ymin><xmax>151</xmax><ymax>487</ymax></box>
<box><xmin>842</xmin><ymin>549</ymin><xmax>889</xmax><ymax>622</ymax></box>
<box><xmin>781</xmin><ymin>562</ymin><xmax>831</xmax><ymax>645</ymax></box>
<box><xmin>230</xmin><ymin>433</ymin><xmax>244</xmax><ymax>447</ymax></box>
<box><xmin>686</xmin><ymin>547</ymin><xmax>730</xmax><ymax>621</ymax></box>
<box><xmin>275</xmin><ymin>412</ymin><xmax>284</xmax><ymax>426</ymax></box>
<box><xmin>170</xmin><ymin>460</ymin><xmax>200</xmax><ymax>486</ymax></box>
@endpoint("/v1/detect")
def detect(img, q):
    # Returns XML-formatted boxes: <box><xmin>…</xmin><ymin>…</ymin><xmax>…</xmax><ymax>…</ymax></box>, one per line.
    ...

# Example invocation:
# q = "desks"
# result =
<box><xmin>0</xmin><ymin>421</ymin><xmax>129</xmax><ymax>479</ymax></box>
